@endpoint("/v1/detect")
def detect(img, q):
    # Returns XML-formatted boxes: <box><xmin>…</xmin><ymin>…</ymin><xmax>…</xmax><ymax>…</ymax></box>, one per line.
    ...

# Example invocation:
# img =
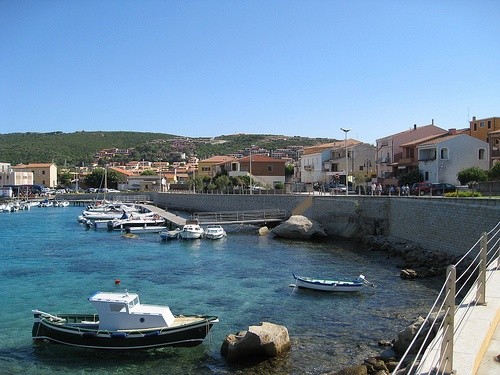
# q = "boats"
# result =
<box><xmin>0</xmin><ymin>198</ymin><xmax>69</xmax><ymax>213</ymax></box>
<box><xmin>290</xmin><ymin>272</ymin><xmax>365</xmax><ymax>291</ymax></box>
<box><xmin>77</xmin><ymin>164</ymin><xmax>168</xmax><ymax>233</ymax></box>
<box><xmin>31</xmin><ymin>290</ymin><xmax>219</xmax><ymax>359</ymax></box>
<box><xmin>204</xmin><ymin>225</ymin><xmax>227</xmax><ymax>240</ymax></box>
<box><xmin>160</xmin><ymin>225</ymin><xmax>204</xmax><ymax>239</ymax></box>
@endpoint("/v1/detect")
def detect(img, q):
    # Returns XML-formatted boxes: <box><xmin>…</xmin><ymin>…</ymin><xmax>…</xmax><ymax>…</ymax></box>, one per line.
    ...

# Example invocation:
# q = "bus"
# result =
<box><xmin>2</xmin><ymin>184</ymin><xmax>45</xmax><ymax>196</ymax></box>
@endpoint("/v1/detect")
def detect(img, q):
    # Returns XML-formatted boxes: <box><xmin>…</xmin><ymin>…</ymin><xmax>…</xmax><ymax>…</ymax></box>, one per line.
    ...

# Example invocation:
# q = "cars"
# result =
<box><xmin>383</xmin><ymin>183</ymin><xmax>396</xmax><ymax>195</ymax></box>
<box><xmin>41</xmin><ymin>186</ymin><xmax>66</xmax><ymax>195</ymax></box>
<box><xmin>411</xmin><ymin>181</ymin><xmax>456</xmax><ymax>196</ymax></box>
<box><xmin>337</xmin><ymin>183</ymin><xmax>348</xmax><ymax>193</ymax></box>
<box><xmin>84</xmin><ymin>188</ymin><xmax>120</xmax><ymax>193</ymax></box>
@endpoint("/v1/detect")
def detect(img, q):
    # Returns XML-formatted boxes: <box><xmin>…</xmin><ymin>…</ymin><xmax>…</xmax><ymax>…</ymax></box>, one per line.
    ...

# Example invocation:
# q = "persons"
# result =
<box><xmin>120</xmin><ymin>211</ymin><xmax>128</xmax><ymax>220</ymax></box>
<box><xmin>376</xmin><ymin>184</ymin><xmax>382</xmax><ymax>195</ymax></box>
<box><xmin>371</xmin><ymin>182</ymin><xmax>376</xmax><ymax>195</ymax></box>
<box><xmin>391</xmin><ymin>184</ymin><xmax>409</xmax><ymax>196</ymax></box>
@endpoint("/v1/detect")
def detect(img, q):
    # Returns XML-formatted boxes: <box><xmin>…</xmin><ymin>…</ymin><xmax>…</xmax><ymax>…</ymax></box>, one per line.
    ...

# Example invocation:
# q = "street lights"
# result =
<box><xmin>340</xmin><ymin>128</ymin><xmax>352</xmax><ymax>195</ymax></box>
<box><xmin>244</xmin><ymin>148</ymin><xmax>256</xmax><ymax>194</ymax></box>
<box><xmin>158</xmin><ymin>157</ymin><xmax>162</xmax><ymax>193</ymax></box>
<box><xmin>74</xmin><ymin>166</ymin><xmax>79</xmax><ymax>194</ymax></box>
<box><xmin>363</xmin><ymin>158</ymin><xmax>372</xmax><ymax>194</ymax></box>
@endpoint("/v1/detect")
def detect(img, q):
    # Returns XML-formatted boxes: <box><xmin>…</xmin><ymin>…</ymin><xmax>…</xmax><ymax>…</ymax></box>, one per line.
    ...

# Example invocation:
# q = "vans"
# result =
<box><xmin>0</xmin><ymin>186</ymin><xmax>13</xmax><ymax>198</ymax></box>
